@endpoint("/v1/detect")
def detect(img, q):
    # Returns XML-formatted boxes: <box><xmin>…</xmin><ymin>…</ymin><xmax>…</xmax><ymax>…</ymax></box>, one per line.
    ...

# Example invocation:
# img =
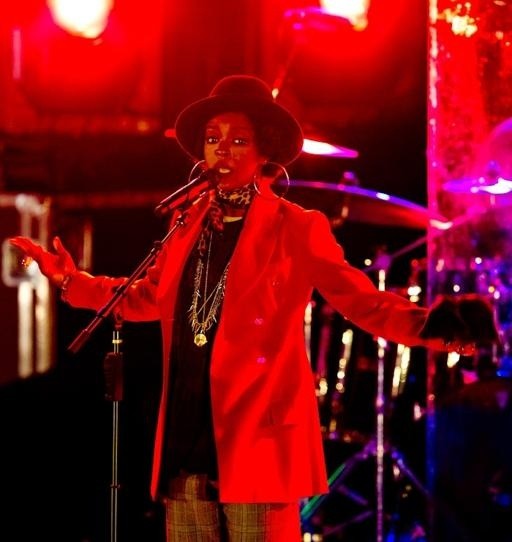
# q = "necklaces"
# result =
<box><xmin>187</xmin><ymin>210</ymin><xmax>231</xmax><ymax>348</ymax></box>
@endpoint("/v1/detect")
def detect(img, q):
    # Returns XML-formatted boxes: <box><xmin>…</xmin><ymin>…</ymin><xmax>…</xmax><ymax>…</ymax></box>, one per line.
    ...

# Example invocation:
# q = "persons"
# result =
<box><xmin>7</xmin><ymin>74</ymin><xmax>501</xmax><ymax>541</ymax></box>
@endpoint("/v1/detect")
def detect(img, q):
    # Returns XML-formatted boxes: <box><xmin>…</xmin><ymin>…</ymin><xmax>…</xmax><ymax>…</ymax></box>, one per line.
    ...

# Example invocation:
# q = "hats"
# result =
<box><xmin>174</xmin><ymin>74</ymin><xmax>304</xmax><ymax>177</ymax></box>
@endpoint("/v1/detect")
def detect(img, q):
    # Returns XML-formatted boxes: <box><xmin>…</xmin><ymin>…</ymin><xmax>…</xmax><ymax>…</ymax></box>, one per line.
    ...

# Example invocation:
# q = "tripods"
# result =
<box><xmin>300</xmin><ymin>250</ymin><xmax>431</xmax><ymax>542</ymax></box>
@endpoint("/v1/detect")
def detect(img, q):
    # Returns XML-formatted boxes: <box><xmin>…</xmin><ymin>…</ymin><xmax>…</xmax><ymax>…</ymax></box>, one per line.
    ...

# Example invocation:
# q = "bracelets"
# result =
<box><xmin>58</xmin><ymin>267</ymin><xmax>78</xmax><ymax>292</ymax></box>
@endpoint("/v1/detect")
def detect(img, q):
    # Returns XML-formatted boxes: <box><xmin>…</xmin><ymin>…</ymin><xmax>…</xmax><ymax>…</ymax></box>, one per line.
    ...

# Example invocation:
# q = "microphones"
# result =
<box><xmin>154</xmin><ymin>169</ymin><xmax>221</xmax><ymax>218</ymax></box>
